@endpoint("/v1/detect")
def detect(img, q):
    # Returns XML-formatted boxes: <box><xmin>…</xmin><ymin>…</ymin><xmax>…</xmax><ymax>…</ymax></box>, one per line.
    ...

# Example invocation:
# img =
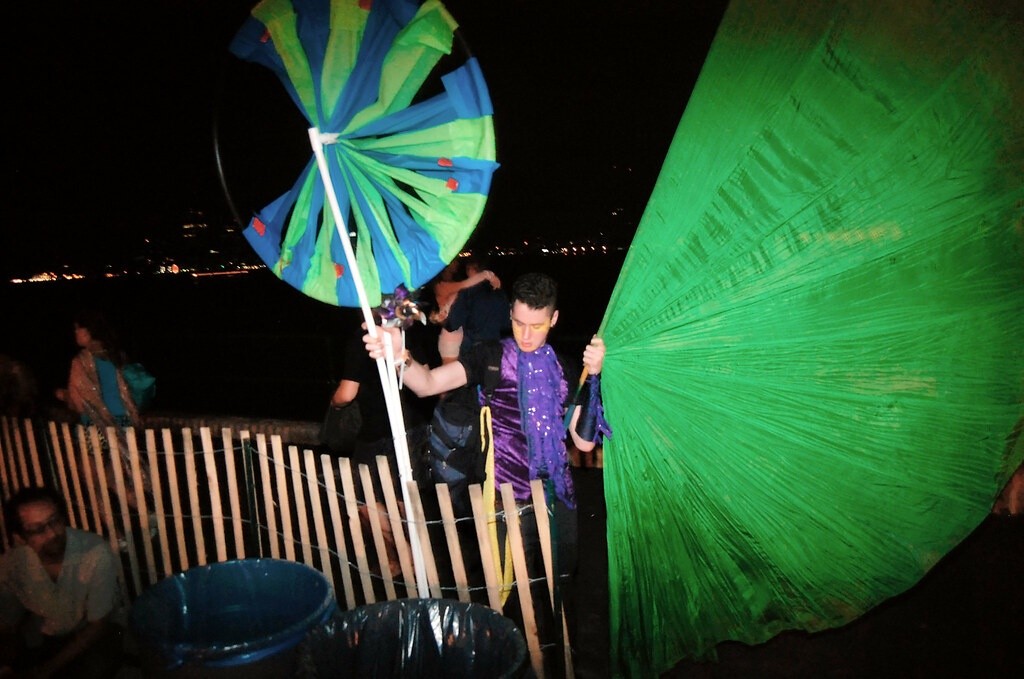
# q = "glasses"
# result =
<box><xmin>25</xmin><ymin>512</ymin><xmax>64</xmax><ymax>536</ymax></box>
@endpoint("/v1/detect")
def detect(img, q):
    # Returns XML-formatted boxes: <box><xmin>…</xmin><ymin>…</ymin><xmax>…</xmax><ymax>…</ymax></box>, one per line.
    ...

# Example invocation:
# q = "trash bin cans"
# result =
<box><xmin>312</xmin><ymin>598</ymin><xmax>527</xmax><ymax>679</ymax></box>
<box><xmin>131</xmin><ymin>559</ymin><xmax>339</xmax><ymax>679</ymax></box>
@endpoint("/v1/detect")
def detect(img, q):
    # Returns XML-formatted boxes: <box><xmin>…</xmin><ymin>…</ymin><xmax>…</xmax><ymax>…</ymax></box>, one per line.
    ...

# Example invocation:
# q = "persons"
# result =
<box><xmin>56</xmin><ymin>314</ymin><xmax>160</xmax><ymax>551</ymax></box>
<box><xmin>326</xmin><ymin>256</ymin><xmax>606</xmax><ymax>678</ymax></box>
<box><xmin>0</xmin><ymin>487</ymin><xmax>127</xmax><ymax>679</ymax></box>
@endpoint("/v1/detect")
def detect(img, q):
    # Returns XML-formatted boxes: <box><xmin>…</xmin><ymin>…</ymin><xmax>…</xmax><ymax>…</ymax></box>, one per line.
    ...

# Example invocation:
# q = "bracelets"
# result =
<box><xmin>395</xmin><ymin>349</ymin><xmax>413</xmax><ymax>374</ymax></box>
<box><xmin>485</xmin><ymin>270</ymin><xmax>494</xmax><ymax>281</ymax></box>
